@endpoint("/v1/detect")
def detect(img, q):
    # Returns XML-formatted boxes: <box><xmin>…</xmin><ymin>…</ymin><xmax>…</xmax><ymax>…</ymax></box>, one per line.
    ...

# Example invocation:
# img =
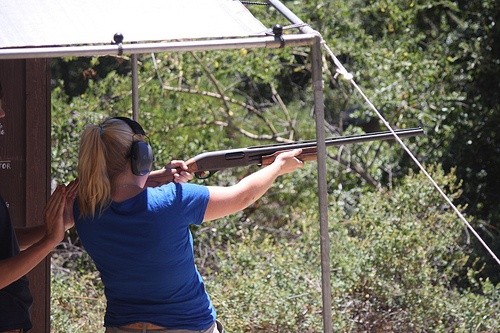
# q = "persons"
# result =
<box><xmin>69</xmin><ymin>116</ymin><xmax>310</xmax><ymax>333</ymax></box>
<box><xmin>0</xmin><ymin>104</ymin><xmax>82</xmax><ymax>332</ymax></box>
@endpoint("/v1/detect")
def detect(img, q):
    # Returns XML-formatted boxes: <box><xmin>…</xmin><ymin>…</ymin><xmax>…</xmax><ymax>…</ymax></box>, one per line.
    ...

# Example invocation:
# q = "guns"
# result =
<box><xmin>144</xmin><ymin>125</ymin><xmax>425</xmax><ymax>186</ymax></box>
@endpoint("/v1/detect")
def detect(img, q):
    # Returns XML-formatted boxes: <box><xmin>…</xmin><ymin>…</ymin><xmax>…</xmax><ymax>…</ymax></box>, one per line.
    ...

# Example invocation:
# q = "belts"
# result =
<box><xmin>123</xmin><ymin>321</ymin><xmax>161</xmax><ymax>330</ymax></box>
